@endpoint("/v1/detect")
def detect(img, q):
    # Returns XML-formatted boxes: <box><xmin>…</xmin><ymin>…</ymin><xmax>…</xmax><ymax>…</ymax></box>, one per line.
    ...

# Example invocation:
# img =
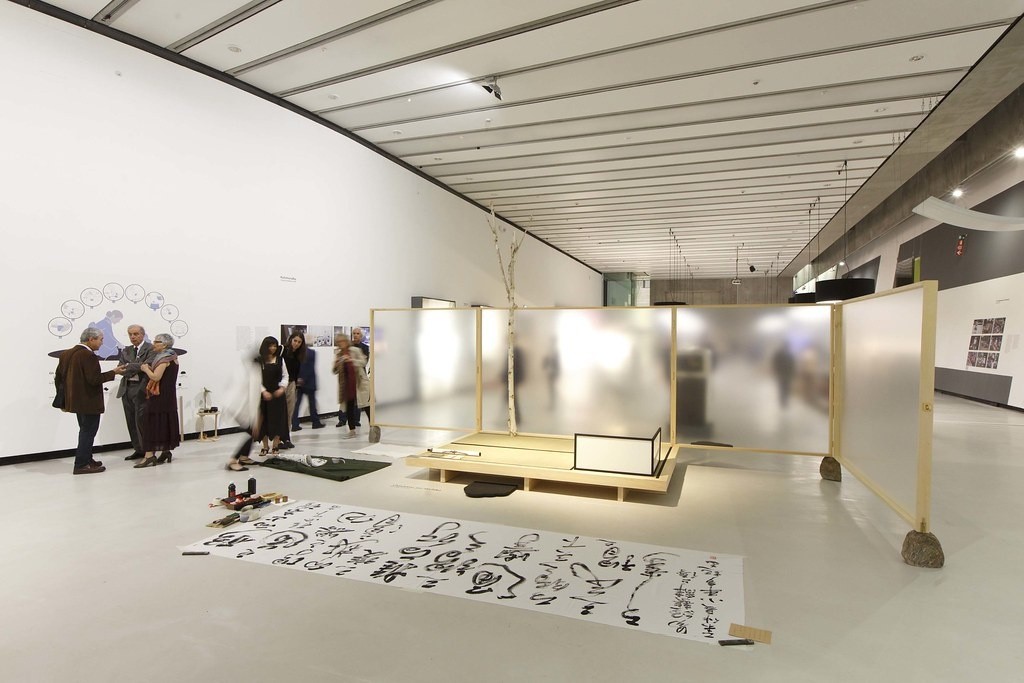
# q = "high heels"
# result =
<box><xmin>259</xmin><ymin>447</ymin><xmax>270</xmax><ymax>456</ymax></box>
<box><xmin>272</xmin><ymin>447</ymin><xmax>279</xmax><ymax>455</ymax></box>
<box><xmin>134</xmin><ymin>456</ymin><xmax>156</xmax><ymax>468</ymax></box>
<box><xmin>157</xmin><ymin>452</ymin><xmax>172</xmax><ymax>464</ymax></box>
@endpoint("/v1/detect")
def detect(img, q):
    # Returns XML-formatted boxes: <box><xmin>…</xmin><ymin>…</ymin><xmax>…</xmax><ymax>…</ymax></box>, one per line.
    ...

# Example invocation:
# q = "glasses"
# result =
<box><xmin>153</xmin><ymin>340</ymin><xmax>162</xmax><ymax>344</ymax></box>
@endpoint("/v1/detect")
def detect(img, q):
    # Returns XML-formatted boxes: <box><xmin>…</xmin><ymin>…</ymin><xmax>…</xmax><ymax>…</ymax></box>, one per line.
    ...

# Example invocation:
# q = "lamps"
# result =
<box><xmin>788</xmin><ymin>161</ymin><xmax>875</xmax><ymax>305</ymax></box>
<box><xmin>481</xmin><ymin>78</ymin><xmax>501</xmax><ymax>99</ymax></box>
<box><xmin>730</xmin><ymin>248</ymin><xmax>741</xmax><ymax>284</ymax></box>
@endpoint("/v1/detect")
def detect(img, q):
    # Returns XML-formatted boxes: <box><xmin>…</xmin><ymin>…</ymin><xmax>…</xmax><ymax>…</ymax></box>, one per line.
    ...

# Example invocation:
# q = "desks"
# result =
<box><xmin>198</xmin><ymin>412</ymin><xmax>222</xmax><ymax>441</ymax></box>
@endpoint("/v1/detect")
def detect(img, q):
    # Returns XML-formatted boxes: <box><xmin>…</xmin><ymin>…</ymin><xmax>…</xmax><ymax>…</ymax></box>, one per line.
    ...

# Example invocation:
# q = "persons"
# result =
<box><xmin>225</xmin><ymin>332</ymin><xmax>307</xmax><ymax>471</ymax></box>
<box><xmin>333</xmin><ymin>333</ymin><xmax>376</xmax><ymax>438</ymax></box>
<box><xmin>116</xmin><ymin>324</ymin><xmax>157</xmax><ymax>460</ymax></box>
<box><xmin>541</xmin><ymin>338</ymin><xmax>562</xmax><ymax>407</ymax></box>
<box><xmin>335</xmin><ymin>328</ymin><xmax>370</xmax><ymax>427</ymax></box>
<box><xmin>53</xmin><ymin>328</ymin><xmax>127</xmax><ymax>474</ymax></box>
<box><xmin>502</xmin><ymin>335</ymin><xmax>525</xmax><ymax>424</ymax></box>
<box><xmin>290</xmin><ymin>334</ymin><xmax>326</xmax><ymax>431</ymax></box>
<box><xmin>771</xmin><ymin>343</ymin><xmax>794</xmax><ymax>406</ymax></box>
<box><xmin>134</xmin><ymin>334</ymin><xmax>180</xmax><ymax>467</ymax></box>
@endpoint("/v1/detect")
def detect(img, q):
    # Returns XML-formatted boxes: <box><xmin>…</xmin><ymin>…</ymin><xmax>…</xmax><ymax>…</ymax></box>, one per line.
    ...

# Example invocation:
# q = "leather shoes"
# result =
<box><xmin>89</xmin><ymin>459</ymin><xmax>102</xmax><ymax>467</ymax></box>
<box><xmin>73</xmin><ymin>464</ymin><xmax>106</xmax><ymax>474</ymax></box>
<box><xmin>125</xmin><ymin>450</ymin><xmax>145</xmax><ymax>460</ymax></box>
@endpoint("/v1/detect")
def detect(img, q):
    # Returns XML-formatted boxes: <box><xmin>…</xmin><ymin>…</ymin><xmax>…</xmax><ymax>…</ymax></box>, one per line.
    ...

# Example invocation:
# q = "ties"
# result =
<box><xmin>131</xmin><ymin>346</ymin><xmax>138</xmax><ymax>378</ymax></box>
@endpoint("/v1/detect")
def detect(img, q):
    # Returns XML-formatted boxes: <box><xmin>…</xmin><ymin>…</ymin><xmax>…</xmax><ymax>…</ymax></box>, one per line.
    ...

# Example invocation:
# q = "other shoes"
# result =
<box><xmin>312</xmin><ymin>424</ymin><xmax>325</xmax><ymax>429</ymax></box>
<box><xmin>355</xmin><ymin>421</ymin><xmax>362</xmax><ymax>426</ymax></box>
<box><xmin>237</xmin><ymin>458</ymin><xmax>260</xmax><ymax>465</ymax></box>
<box><xmin>336</xmin><ymin>421</ymin><xmax>346</xmax><ymax>427</ymax></box>
<box><xmin>226</xmin><ymin>463</ymin><xmax>248</xmax><ymax>471</ymax></box>
<box><xmin>284</xmin><ymin>440</ymin><xmax>295</xmax><ymax>448</ymax></box>
<box><xmin>343</xmin><ymin>430</ymin><xmax>356</xmax><ymax>439</ymax></box>
<box><xmin>292</xmin><ymin>427</ymin><xmax>302</xmax><ymax>431</ymax></box>
<box><xmin>278</xmin><ymin>442</ymin><xmax>288</xmax><ymax>449</ymax></box>
